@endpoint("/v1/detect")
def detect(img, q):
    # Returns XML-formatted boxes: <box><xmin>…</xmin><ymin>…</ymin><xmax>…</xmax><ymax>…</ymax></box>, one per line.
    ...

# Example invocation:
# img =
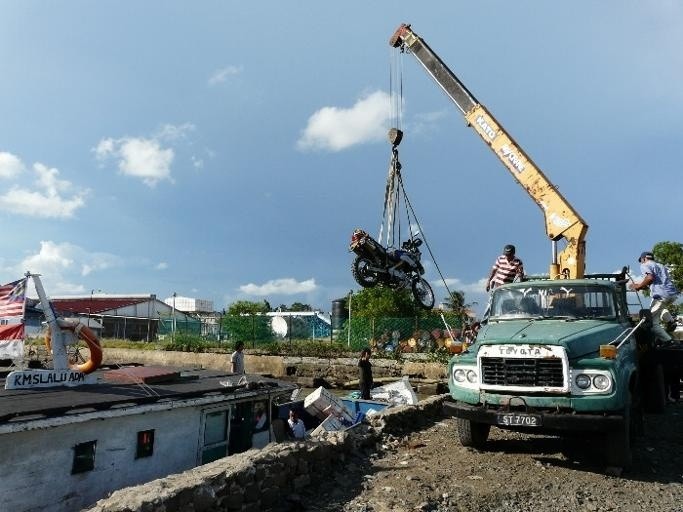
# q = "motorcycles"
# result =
<box><xmin>348</xmin><ymin>227</ymin><xmax>435</xmax><ymax>310</ymax></box>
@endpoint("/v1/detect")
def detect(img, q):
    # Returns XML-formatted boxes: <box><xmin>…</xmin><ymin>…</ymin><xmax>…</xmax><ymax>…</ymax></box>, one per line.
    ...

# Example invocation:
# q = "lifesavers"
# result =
<box><xmin>45</xmin><ymin>324</ymin><xmax>101</xmax><ymax>374</ymax></box>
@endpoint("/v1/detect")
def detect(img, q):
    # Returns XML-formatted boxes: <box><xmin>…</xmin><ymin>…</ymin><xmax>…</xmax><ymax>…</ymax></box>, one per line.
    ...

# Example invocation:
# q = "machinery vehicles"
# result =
<box><xmin>388</xmin><ymin>22</ymin><xmax>667</xmax><ymax>464</ymax></box>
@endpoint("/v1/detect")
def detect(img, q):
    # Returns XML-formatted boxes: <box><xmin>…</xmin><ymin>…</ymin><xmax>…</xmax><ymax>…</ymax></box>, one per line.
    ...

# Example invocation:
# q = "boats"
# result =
<box><xmin>277</xmin><ymin>374</ymin><xmax>419</xmax><ymax>473</ymax></box>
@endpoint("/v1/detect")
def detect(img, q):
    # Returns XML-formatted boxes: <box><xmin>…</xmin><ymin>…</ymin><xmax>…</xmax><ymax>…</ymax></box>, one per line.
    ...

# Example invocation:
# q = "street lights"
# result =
<box><xmin>172</xmin><ymin>291</ymin><xmax>177</xmax><ymax>343</ymax></box>
<box><xmin>347</xmin><ymin>289</ymin><xmax>353</xmax><ymax>347</ymax></box>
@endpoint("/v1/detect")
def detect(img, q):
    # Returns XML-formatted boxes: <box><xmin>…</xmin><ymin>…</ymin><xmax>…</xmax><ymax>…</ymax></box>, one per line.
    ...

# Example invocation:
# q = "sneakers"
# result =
<box><xmin>666</xmin><ymin>321</ymin><xmax>677</xmax><ymax>332</ymax></box>
<box><xmin>662</xmin><ymin>339</ymin><xmax>675</xmax><ymax>347</ymax></box>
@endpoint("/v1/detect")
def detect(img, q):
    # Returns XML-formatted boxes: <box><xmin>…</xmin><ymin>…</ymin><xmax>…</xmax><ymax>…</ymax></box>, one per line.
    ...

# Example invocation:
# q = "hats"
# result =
<box><xmin>503</xmin><ymin>245</ymin><xmax>515</xmax><ymax>255</ymax></box>
<box><xmin>638</xmin><ymin>252</ymin><xmax>654</xmax><ymax>263</ymax></box>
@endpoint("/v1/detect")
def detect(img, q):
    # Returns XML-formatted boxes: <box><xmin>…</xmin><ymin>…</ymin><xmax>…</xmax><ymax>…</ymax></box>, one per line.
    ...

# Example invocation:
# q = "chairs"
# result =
<box><xmin>498</xmin><ymin>297</ymin><xmax>581</xmax><ymax>317</ymax></box>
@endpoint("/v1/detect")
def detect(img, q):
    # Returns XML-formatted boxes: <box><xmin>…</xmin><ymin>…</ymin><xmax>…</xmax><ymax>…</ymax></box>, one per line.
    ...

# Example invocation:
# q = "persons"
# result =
<box><xmin>357</xmin><ymin>348</ymin><xmax>374</xmax><ymax>400</ymax></box>
<box><xmin>627</xmin><ymin>251</ymin><xmax>677</xmax><ymax>346</ymax></box>
<box><xmin>230</xmin><ymin>340</ymin><xmax>246</xmax><ymax>374</ymax></box>
<box><xmin>287</xmin><ymin>408</ymin><xmax>306</xmax><ymax>439</ymax></box>
<box><xmin>480</xmin><ymin>244</ymin><xmax>524</xmax><ymax>327</ymax></box>
